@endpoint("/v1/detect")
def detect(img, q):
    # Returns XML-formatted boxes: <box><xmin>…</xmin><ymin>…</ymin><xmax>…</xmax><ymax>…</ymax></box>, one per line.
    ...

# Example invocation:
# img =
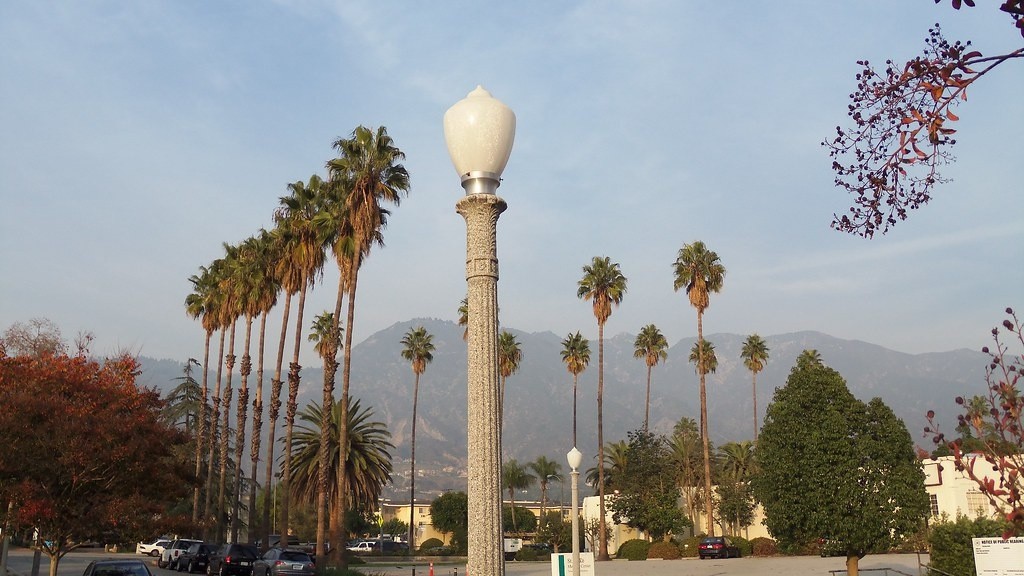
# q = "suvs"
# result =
<box><xmin>158</xmin><ymin>537</ymin><xmax>205</xmax><ymax>570</ymax></box>
<box><xmin>207</xmin><ymin>541</ymin><xmax>261</xmax><ymax>576</ymax></box>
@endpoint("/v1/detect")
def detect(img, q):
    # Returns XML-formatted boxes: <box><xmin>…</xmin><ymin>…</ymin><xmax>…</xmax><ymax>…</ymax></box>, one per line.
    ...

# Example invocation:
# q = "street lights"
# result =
<box><xmin>442</xmin><ymin>83</ymin><xmax>519</xmax><ymax>576</ymax></box>
<box><xmin>566</xmin><ymin>444</ymin><xmax>582</xmax><ymax>576</ymax></box>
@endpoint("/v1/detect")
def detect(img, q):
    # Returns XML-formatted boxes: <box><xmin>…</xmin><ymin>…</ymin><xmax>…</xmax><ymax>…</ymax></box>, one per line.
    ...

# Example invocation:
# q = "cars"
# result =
<box><xmin>346</xmin><ymin>541</ymin><xmax>377</xmax><ymax>553</ymax></box>
<box><xmin>176</xmin><ymin>543</ymin><xmax>219</xmax><ymax>574</ymax></box>
<box><xmin>139</xmin><ymin>539</ymin><xmax>171</xmax><ymax>558</ymax></box>
<box><xmin>82</xmin><ymin>557</ymin><xmax>137</xmax><ymax>576</ymax></box>
<box><xmin>249</xmin><ymin>546</ymin><xmax>316</xmax><ymax>576</ymax></box>
<box><xmin>698</xmin><ymin>535</ymin><xmax>740</xmax><ymax>559</ymax></box>
